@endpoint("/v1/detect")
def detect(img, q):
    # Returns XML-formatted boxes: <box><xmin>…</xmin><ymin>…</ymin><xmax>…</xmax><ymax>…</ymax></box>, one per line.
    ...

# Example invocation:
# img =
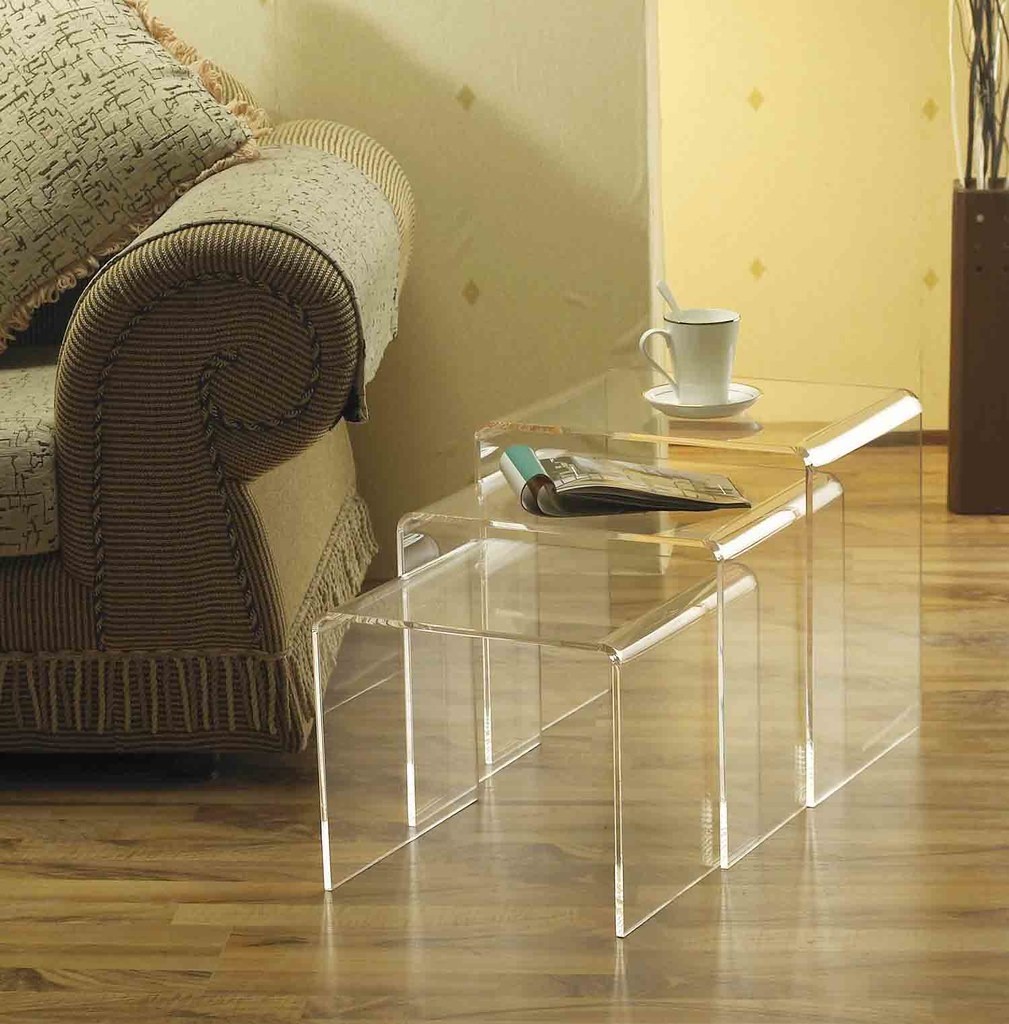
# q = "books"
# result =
<box><xmin>499</xmin><ymin>446</ymin><xmax>752</xmax><ymax>518</ymax></box>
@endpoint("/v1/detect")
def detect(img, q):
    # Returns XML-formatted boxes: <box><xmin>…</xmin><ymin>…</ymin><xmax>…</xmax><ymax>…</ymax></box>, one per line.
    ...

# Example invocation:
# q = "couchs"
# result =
<box><xmin>0</xmin><ymin>121</ymin><xmax>416</xmax><ymax>759</ymax></box>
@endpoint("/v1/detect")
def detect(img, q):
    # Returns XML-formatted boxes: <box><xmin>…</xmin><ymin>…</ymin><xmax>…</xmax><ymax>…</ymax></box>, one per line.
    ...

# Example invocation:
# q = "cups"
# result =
<box><xmin>640</xmin><ymin>308</ymin><xmax>741</xmax><ymax>406</ymax></box>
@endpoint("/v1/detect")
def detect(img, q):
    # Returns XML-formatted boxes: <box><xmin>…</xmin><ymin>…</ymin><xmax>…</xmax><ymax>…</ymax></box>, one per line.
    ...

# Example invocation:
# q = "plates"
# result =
<box><xmin>644</xmin><ymin>384</ymin><xmax>761</xmax><ymax>418</ymax></box>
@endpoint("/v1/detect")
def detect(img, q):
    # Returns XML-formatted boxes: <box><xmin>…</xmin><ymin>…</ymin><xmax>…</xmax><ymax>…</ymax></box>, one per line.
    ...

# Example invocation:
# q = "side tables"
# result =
<box><xmin>309</xmin><ymin>369</ymin><xmax>923</xmax><ymax>941</ymax></box>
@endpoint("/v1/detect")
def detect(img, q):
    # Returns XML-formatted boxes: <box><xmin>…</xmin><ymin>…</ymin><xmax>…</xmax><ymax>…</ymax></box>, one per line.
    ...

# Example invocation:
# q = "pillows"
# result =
<box><xmin>0</xmin><ymin>5</ymin><xmax>269</xmax><ymax>349</ymax></box>
